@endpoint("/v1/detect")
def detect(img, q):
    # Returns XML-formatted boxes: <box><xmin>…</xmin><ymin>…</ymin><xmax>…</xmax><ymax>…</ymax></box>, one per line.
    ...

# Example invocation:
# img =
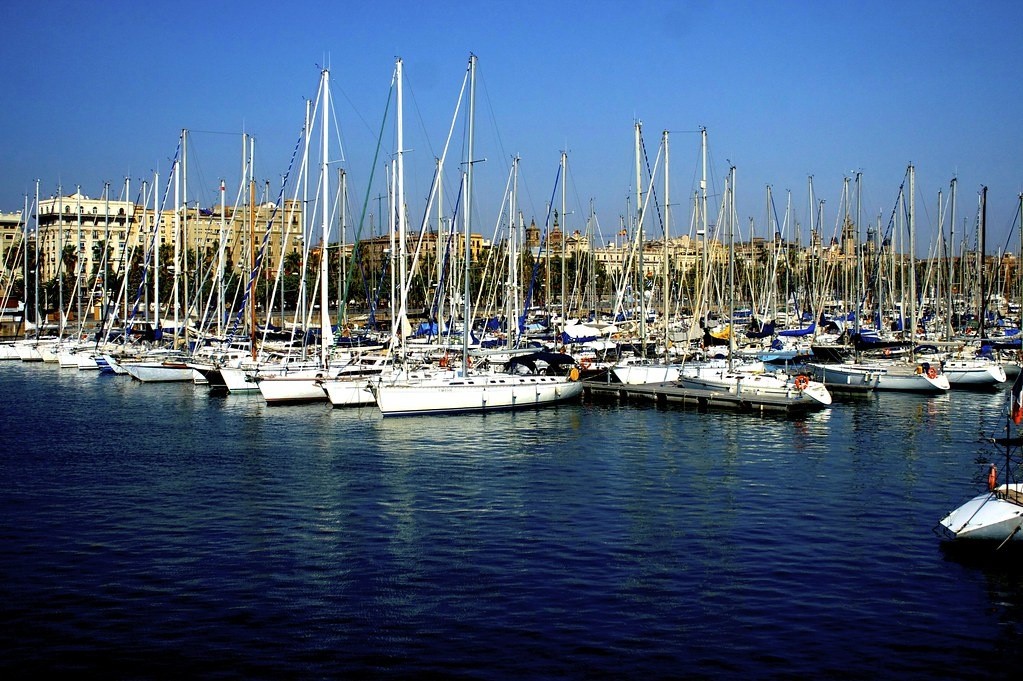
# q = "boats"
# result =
<box><xmin>930</xmin><ymin>388</ymin><xmax>1023</xmax><ymax>551</ymax></box>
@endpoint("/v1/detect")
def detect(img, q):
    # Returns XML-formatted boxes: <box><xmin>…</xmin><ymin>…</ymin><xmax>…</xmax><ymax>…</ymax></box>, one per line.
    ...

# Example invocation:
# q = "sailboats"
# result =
<box><xmin>0</xmin><ymin>51</ymin><xmax>1023</xmax><ymax>429</ymax></box>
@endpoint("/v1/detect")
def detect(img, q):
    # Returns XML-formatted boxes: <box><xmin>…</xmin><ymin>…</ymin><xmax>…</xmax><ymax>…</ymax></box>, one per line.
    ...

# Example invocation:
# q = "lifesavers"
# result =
<box><xmin>582</xmin><ymin>357</ymin><xmax>591</xmax><ymax>368</ymax></box>
<box><xmin>929</xmin><ymin>368</ymin><xmax>936</xmax><ymax>379</ymax></box>
<box><xmin>988</xmin><ymin>465</ymin><xmax>996</xmax><ymax>491</ymax></box>
<box><xmin>795</xmin><ymin>375</ymin><xmax>809</xmax><ymax>390</ymax></box>
<box><xmin>570</xmin><ymin>368</ymin><xmax>579</xmax><ymax>381</ymax></box>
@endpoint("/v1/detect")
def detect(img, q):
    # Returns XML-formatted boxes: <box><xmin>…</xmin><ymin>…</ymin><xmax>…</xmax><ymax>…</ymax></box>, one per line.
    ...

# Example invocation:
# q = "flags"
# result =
<box><xmin>1011</xmin><ymin>367</ymin><xmax>1023</xmax><ymax>425</ymax></box>
